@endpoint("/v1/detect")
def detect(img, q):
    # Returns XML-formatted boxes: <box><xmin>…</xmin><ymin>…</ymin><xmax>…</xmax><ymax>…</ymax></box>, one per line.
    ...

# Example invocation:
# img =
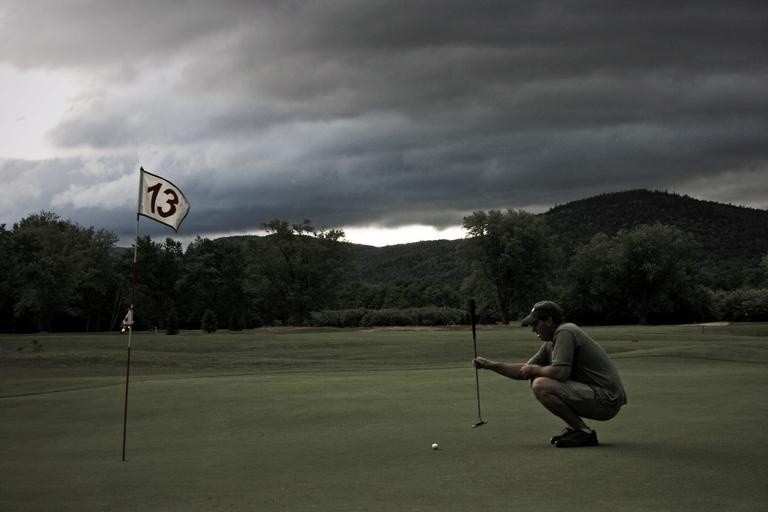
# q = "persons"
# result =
<box><xmin>472</xmin><ymin>301</ymin><xmax>627</xmax><ymax>448</ymax></box>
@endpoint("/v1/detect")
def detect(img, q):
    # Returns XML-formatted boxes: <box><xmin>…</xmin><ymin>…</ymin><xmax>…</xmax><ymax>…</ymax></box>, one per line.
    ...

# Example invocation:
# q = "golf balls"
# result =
<box><xmin>432</xmin><ymin>443</ymin><xmax>438</xmax><ymax>449</ymax></box>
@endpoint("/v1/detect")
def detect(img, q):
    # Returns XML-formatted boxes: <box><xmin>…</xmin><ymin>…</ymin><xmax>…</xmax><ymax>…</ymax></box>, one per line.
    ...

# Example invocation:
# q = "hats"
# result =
<box><xmin>520</xmin><ymin>301</ymin><xmax>563</xmax><ymax>327</ymax></box>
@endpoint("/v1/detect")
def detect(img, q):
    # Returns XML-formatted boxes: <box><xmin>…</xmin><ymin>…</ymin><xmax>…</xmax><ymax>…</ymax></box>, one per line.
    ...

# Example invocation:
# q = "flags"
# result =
<box><xmin>140</xmin><ymin>170</ymin><xmax>190</xmax><ymax>234</ymax></box>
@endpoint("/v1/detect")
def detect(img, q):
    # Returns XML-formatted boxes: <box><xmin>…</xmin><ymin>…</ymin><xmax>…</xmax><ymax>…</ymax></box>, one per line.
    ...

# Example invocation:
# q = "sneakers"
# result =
<box><xmin>550</xmin><ymin>427</ymin><xmax>600</xmax><ymax>448</ymax></box>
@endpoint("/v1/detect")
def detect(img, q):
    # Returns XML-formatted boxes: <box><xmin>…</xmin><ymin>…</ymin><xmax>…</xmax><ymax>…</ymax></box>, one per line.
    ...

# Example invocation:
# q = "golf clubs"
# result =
<box><xmin>470</xmin><ymin>299</ymin><xmax>487</xmax><ymax>428</ymax></box>
